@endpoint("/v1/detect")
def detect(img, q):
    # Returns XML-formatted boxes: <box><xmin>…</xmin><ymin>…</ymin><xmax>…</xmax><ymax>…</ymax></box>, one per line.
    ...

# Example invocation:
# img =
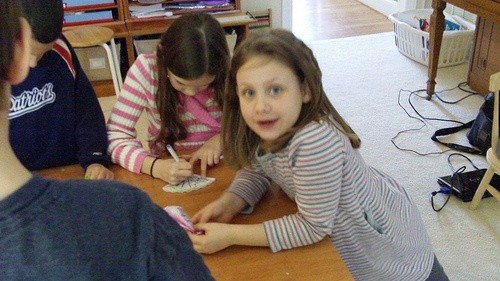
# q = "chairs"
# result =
<box><xmin>469</xmin><ymin>71</ymin><xmax>500</xmax><ymax>211</ymax></box>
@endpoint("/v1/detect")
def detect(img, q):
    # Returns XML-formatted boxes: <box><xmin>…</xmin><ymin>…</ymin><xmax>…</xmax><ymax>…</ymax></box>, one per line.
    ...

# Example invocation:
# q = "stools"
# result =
<box><xmin>63</xmin><ymin>27</ymin><xmax>123</xmax><ymax>97</ymax></box>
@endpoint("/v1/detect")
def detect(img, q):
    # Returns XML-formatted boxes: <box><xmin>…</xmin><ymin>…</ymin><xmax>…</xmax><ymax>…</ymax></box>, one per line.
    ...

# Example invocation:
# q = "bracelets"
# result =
<box><xmin>150</xmin><ymin>157</ymin><xmax>161</xmax><ymax>178</ymax></box>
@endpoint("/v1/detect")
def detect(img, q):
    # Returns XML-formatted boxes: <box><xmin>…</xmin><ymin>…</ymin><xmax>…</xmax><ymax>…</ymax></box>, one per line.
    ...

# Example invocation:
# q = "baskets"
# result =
<box><xmin>388</xmin><ymin>8</ymin><xmax>477</xmax><ymax>68</ymax></box>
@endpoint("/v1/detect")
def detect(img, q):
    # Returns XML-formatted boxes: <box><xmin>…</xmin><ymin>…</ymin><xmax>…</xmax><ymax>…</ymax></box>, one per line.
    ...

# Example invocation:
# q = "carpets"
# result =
<box><xmin>92</xmin><ymin>30</ymin><xmax>500</xmax><ymax>281</ymax></box>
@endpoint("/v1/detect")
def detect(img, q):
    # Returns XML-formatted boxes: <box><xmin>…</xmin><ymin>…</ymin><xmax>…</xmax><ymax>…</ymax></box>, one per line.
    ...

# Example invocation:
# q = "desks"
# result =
<box><xmin>33</xmin><ymin>149</ymin><xmax>355</xmax><ymax>281</ymax></box>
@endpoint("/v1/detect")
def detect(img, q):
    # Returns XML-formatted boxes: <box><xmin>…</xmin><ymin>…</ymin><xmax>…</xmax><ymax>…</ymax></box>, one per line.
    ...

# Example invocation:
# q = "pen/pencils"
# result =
<box><xmin>166</xmin><ymin>144</ymin><xmax>180</xmax><ymax>162</ymax></box>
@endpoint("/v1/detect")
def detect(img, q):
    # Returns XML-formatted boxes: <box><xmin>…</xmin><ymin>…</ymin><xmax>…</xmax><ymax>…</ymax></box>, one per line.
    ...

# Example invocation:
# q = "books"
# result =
<box><xmin>128</xmin><ymin>0</ymin><xmax>173</xmax><ymax>18</ymax></box>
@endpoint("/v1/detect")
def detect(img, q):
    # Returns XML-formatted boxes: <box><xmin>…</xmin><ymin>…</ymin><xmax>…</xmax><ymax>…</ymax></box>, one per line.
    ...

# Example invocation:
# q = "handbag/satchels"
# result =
<box><xmin>467</xmin><ymin>93</ymin><xmax>500</xmax><ymax>151</ymax></box>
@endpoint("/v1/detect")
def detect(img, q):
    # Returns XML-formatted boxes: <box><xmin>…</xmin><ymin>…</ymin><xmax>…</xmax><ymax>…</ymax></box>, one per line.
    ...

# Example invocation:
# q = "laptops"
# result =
<box><xmin>437</xmin><ymin>169</ymin><xmax>500</xmax><ymax>202</ymax></box>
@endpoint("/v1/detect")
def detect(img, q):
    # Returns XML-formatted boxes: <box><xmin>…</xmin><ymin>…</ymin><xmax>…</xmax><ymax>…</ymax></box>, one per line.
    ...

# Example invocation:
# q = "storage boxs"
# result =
<box><xmin>389</xmin><ymin>8</ymin><xmax>475</xmax><ymax>68</ymax></box>
<box><xmin>73</xmin><ymin>38</ymin><xmax>121</xmax><ymax>81</ymax></box>
<box><xmin>133</xmin><ymin>29</ymin><xmax>237</xmax><ymax>55</ymax></box>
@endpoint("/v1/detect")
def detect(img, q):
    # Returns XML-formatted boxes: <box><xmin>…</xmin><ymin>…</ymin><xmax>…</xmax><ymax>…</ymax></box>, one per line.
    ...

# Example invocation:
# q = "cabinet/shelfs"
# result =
<box><xmin>62</xmin><ymin>0</ymin><xmax>258</xmax><ymax>98</ymax></box>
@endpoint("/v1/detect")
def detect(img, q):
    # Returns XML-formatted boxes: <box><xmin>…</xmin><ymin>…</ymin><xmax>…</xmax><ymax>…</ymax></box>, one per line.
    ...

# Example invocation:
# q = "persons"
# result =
<box><xmin>106</xmin><ymin>13</ymin><xmax>230</xmax><ymax>185</ymax></box>
<box><xmin>0</xmin><ymin>0</ymin><xmax>216</xmax><ymax>281</ymax></box>
<box><xmin>187</xmin><ymin>29</ymin><xmax>450</xmax><ymax>281</ymax></box>
<box><xmin>10</xmin><ymin>0</ymin><xmax>115</xmax><ymax>181</ymax></box>
